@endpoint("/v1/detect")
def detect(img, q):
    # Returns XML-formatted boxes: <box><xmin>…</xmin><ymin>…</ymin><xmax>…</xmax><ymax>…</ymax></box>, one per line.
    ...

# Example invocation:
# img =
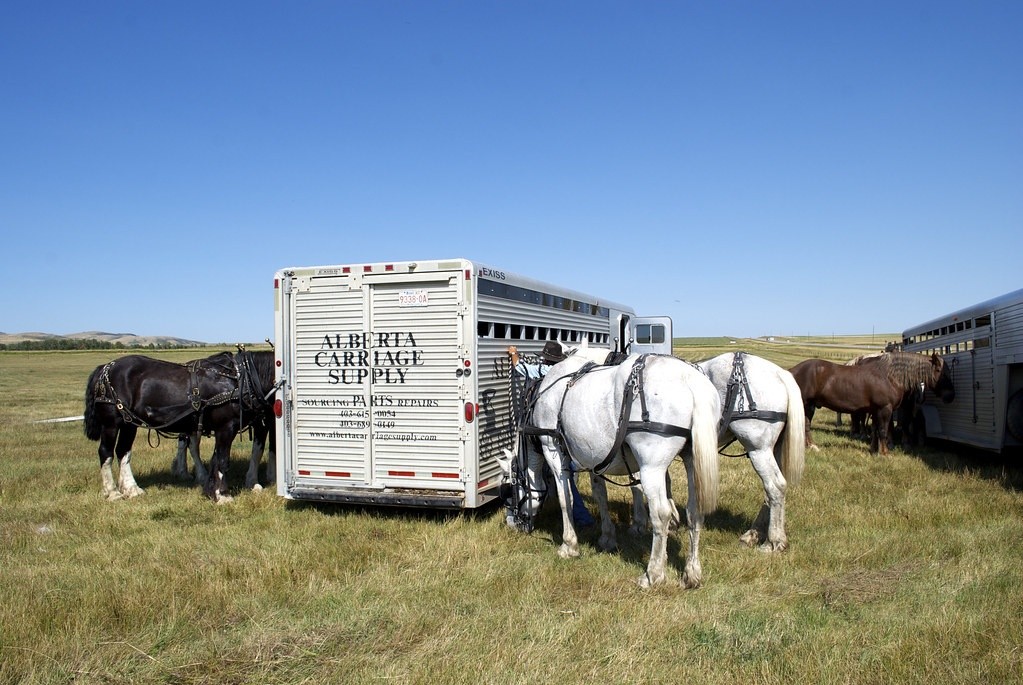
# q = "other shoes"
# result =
<box><xmin>507</xmin><ymin>515</ymin><xmax>535</xmax><ymax>533</ymax></box>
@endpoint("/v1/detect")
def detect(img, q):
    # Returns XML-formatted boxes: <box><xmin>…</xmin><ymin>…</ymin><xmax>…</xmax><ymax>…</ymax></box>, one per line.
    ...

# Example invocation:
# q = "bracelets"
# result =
<box><xmin>510</xmin><ymin>352</ymin><xmax>516</xmax><ymax>356</ymax></box>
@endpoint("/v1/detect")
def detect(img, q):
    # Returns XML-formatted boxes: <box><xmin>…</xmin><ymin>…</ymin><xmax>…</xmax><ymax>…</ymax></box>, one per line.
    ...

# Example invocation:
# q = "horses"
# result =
<box><xmin>80</xmin><ymin>348</ymin><xmax>276</xmax><ymax>507</ymax></box>
<box><xmin>492</xmin><ymin>335</ymin><xmax>957</xmax><ymax>594</ymax></box>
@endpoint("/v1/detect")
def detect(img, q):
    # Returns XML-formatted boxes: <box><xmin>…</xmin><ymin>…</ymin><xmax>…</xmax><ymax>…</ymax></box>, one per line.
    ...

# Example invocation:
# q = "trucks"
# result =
<box><xmin>882</xmin><ymin>289</ymin><xmax>1023</xmax><ymax>477</ymax></box>
<box><xmin>274</xmin><ymin>259</ymin><xmax>672</xmax><ymax>524</ymax></box>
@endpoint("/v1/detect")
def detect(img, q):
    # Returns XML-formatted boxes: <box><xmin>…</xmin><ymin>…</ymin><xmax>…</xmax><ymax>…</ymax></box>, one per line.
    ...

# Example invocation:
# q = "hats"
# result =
<box><xmin>534</xmin><ymin>341</ymin><xmax>568</xmax><ymax>362</ymax></box>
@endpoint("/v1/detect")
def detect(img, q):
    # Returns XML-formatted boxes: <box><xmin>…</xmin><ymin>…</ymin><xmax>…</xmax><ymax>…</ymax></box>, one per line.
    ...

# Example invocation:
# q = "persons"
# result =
<box><xmin>505</xmin><ymin>341</ymin><xmax>568</xmax><ymax>533</ymax></box>
